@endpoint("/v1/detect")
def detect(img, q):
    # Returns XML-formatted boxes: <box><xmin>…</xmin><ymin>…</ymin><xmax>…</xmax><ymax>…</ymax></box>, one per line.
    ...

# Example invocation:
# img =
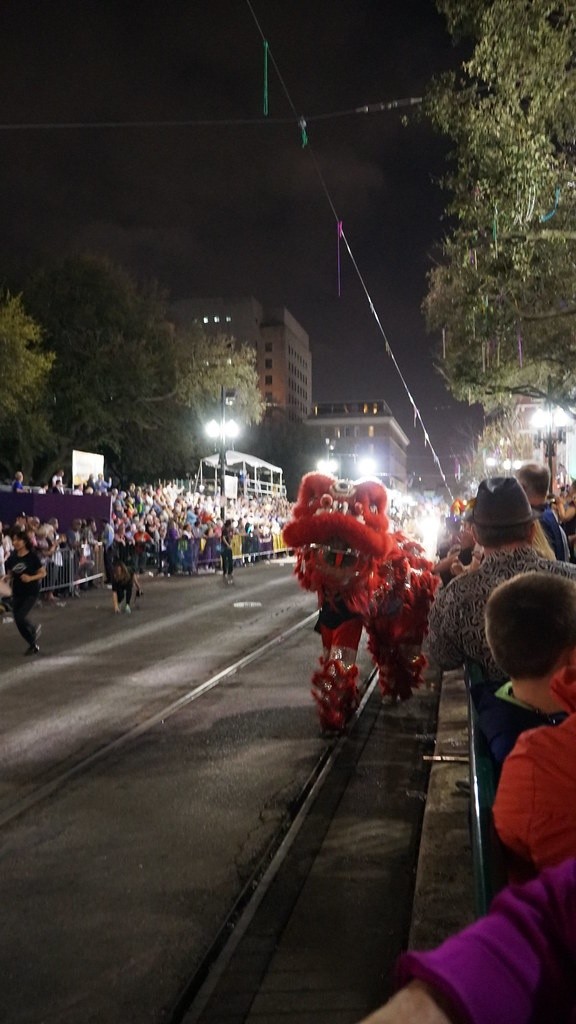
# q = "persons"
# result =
<box><xmin>0</xmin><ymin>468</ymin><xmax>293</xmax><ymax>656</ymax></box>
<box><xmin>360</xmin><ymin>461</ymin><xmax>576</xmax><ymax>1024</ymax></box>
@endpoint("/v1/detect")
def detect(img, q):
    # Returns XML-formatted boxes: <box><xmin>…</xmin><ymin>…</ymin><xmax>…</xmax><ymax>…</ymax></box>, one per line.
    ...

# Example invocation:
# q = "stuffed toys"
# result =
<box><xmin>281</xmin><ymin>471</ymin><xmax>442</xmax><ymax>738</ymax></box>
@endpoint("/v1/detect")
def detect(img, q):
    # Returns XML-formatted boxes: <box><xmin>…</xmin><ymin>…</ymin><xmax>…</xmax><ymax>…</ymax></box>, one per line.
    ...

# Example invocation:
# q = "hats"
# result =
<box><xmin>463</xmin><ymin>476</ymin><xmax>544</xmax><ymax>528</ymax></box>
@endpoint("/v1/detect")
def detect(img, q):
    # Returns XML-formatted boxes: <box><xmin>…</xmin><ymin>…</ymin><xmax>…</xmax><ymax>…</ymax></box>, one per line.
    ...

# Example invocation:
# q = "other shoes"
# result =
<box><xmin>24</xmin><ymin>623</ymin><xmax>42</xmax><ymax>655</ymax></box>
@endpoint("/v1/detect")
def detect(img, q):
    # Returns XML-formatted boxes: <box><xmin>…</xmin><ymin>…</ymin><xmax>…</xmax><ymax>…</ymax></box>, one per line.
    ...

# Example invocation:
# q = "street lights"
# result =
<box><xmin>219</xmin><ymin>382</ymin><xmax>237</xmax><ymax>521</ymax></box>
<box><xmin>531</xmin><ymin>373</ymin><xmax>555</xmax><ymax>502</ymax></box>
